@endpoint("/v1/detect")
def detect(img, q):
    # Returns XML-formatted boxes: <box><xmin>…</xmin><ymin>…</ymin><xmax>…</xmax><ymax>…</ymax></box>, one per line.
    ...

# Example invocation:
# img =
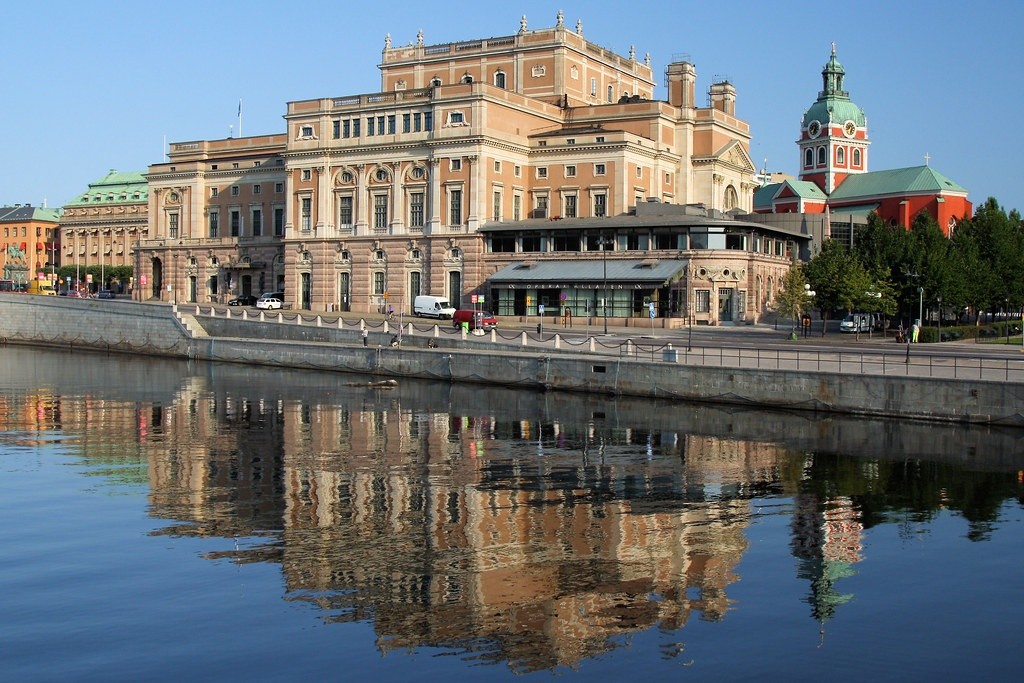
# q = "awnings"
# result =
<box><xmin>486</xmin><ymin>260</ymin><xmax>689</xmax><ymax>289</ymax></box>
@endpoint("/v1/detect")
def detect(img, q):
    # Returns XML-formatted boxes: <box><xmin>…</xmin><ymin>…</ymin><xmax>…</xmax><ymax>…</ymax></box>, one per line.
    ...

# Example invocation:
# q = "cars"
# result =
<box><xmin>229</xmin><ymin>295</ymin><xmax>259</xmax><ymax>306</ymax></box>
<box><xmin>256</xmin><ymin>292</ymin><xmax>284</xmax><ymax>309</ymax></box>
<box><xmin>99</xmin><ymin>290</ymin><xmax>115</xmax><ymax>298</ymax></box>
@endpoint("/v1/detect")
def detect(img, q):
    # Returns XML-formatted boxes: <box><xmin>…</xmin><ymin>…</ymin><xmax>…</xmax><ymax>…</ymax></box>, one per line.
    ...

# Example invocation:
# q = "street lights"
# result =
<box><xmin>596</xmin><ymin>238</ymin><xmax>616</xmax><ymax>334</ymax></box>
<box><xmin>803</xmin><ymin>284</ymin><xmax>816</xmax><ymax>339</ymax></box>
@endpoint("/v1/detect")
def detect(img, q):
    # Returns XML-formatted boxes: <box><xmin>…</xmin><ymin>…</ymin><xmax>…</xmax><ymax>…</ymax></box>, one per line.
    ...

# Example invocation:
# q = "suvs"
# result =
<box><xmin>414</xmin><ymin>295</ymin><xmax>456</xmax><ymax>318</ymax></box>
<box><xmin>840</xmin><ymin>313</ymin><xmax>874</xmax><ymax>333</ymax></box>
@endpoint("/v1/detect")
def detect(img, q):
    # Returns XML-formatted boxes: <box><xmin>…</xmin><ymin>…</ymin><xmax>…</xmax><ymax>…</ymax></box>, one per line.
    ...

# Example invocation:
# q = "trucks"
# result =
<box><xmin>28</xmin><ymin>280</ymin><xmax>56</xmax><ymax>295</ymax></box>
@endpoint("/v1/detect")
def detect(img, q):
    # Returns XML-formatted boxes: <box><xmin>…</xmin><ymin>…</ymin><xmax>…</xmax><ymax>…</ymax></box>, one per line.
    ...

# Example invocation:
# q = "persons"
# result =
<box><xmin>428</xmin><ymin>338</ymin><xmax>435</xmax><ymax>349</ymax></box>
<box><xmin>911</xmin><ymin>322</ymin><xmax>919</xmax><ymax>343</ymax></box>
<box><xmin>362</xmin><ymin>327</ymin><xmax>368</xmax><ymax>347</ymax></box>
<box><xmin>391</xmin><ymin>335</ymin><xmax>398</xmax><ymax>347</ymax></box>
<box><xmin>389</xmin><ymin>305</ymin><xmax>394</xmax><ymax>314</ymax></box>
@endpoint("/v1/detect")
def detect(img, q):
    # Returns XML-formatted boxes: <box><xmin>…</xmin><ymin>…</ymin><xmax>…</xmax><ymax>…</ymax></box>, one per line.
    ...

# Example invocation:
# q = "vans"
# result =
<box><xmin>452</xmin><ymin>310</ymin><xmax>497</xmax><ymax>331</ymax></box>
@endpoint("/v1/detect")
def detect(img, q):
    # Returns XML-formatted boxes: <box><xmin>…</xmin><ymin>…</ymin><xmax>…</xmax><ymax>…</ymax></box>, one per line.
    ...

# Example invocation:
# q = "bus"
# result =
<box><xmin>0</xmin><ymin>280</ymin><xmax>16</xmax><ymax>291</ymax></box>
